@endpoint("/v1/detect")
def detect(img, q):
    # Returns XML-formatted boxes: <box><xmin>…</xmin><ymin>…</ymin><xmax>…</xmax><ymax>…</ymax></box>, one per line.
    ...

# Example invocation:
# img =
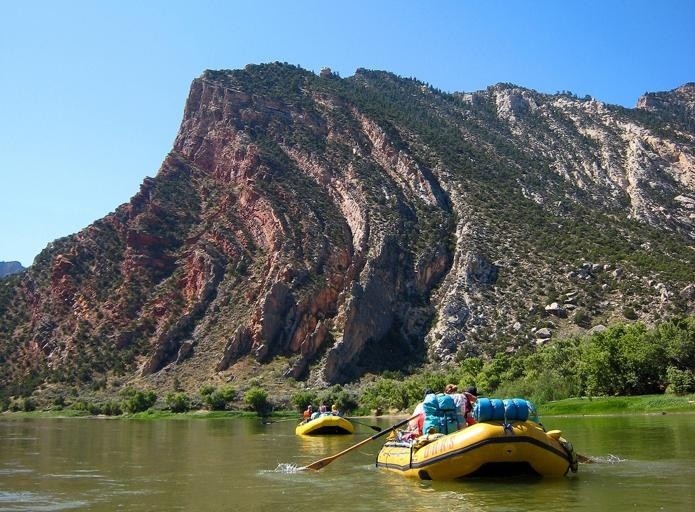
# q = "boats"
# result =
<box><xmin>376</xmin><ymin>429</ymin><xmax>578</xmax><ymax>481</ymax></box>
<box><xmin>295</xmin><ymin>416</ymin><xmax>353</xmax><ymax>436</ymax></box>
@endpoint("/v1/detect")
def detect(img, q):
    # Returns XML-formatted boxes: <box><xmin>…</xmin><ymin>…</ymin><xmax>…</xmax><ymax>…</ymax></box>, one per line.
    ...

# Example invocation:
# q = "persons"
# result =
<box><xmin>306</xmin><ymin>404</ymin><xmax>340</xmax><ymax>419</ymax></box>
<box><xmin>400</xmin><ymin>383</ymin><xmax>479</xmax><ymax>439</ymax></box>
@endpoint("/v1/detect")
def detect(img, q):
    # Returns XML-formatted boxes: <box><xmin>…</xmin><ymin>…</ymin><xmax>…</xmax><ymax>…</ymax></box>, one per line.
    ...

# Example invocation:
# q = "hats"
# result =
<box><xmin>468</xmin><ymin>386</ymin><xmax>483</xmax><ymax>395</ymax></box>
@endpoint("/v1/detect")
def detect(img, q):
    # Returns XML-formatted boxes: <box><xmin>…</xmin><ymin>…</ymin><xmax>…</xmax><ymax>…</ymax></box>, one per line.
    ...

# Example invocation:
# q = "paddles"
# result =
<box><xmin>296</xmin><ymin>412</ymin><xmax>421</xmax><ymax>471</ymax></box>
<box><xmin>573</xmin><ymin>451</ymin><xmax>594</xmax><ymax>463</ymax></box>
<box><xmin>339</xmin><ymin>415</ymin><xmax>382</xmax><ymax>431</ymax></box>
